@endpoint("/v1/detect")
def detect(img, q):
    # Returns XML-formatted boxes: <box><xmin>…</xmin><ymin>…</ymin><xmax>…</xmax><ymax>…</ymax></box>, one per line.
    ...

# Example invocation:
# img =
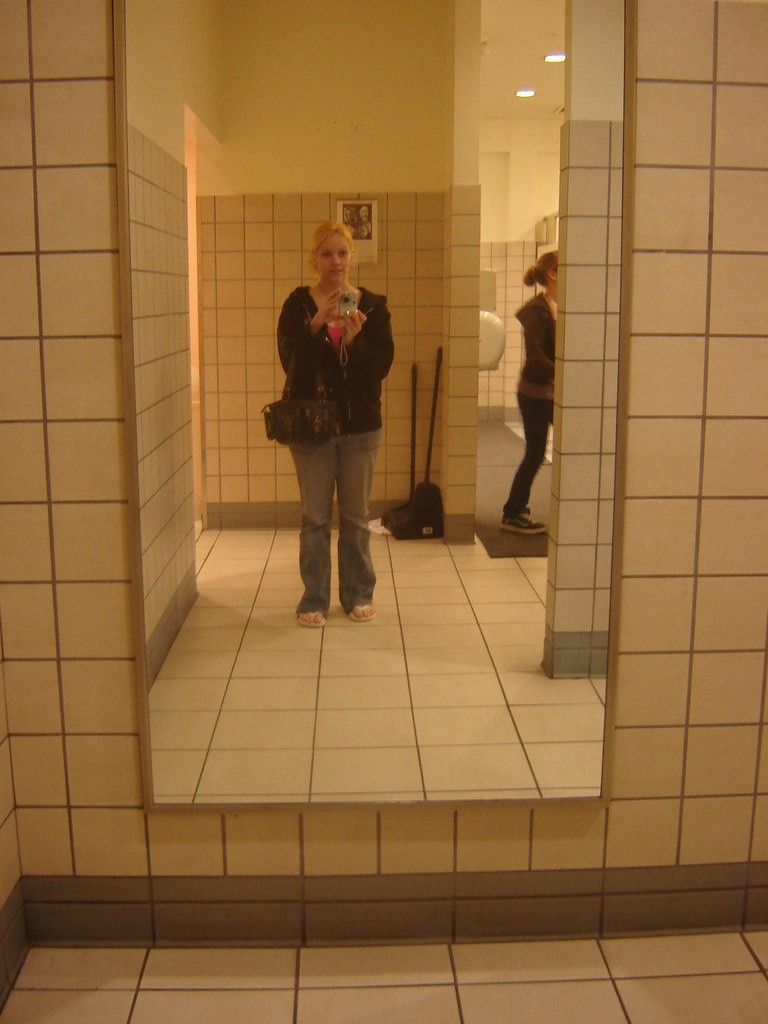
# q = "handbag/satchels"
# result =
<box><xmin>262</xmin><ymin>387</ymin><xmax>338</xmax><ymax>448</ymax></box>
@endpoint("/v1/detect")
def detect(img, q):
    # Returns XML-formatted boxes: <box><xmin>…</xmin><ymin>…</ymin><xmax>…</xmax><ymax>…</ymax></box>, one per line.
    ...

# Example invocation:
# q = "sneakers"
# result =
<box><xmin>500</xmin><ymin>510</ymin><xmax>545</xmax><ymax>535</ymax></box>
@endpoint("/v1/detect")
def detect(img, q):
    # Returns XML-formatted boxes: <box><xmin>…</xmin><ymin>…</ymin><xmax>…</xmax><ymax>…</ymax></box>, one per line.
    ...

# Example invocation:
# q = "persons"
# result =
<box><xmin>499</xmin><ymin>253</ymin><xmax>556</xmax><ymax>536</ymax></box>
<box><xmin>277</xmin><ymin>219</ymin><xmax>395</xmax><ymax>629</ymax></box>
<box><xmin>341</xmin><ymin>205</ymin><xmax>370</xmax><ymax>239</ymax></box>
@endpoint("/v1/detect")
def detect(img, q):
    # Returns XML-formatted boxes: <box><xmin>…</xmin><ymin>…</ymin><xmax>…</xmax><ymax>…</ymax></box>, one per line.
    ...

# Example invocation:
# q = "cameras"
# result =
<box><xmin>340</xmin><ymin>290</ymin><xmax>356</xmax><ymax>317</ymax></box>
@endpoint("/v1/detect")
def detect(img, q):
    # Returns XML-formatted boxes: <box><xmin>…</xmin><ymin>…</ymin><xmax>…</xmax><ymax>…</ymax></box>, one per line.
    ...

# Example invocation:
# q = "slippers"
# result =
<box><xmin>297</xmin><ymin>604</ymin><xmax>376</xmax><ymax>628</ymax></box>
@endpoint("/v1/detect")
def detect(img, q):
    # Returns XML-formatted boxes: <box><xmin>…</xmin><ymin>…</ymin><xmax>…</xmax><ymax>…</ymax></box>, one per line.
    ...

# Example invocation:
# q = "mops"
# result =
<box><xmin>400</xmin><ymin>348</ymin><xmax>445</xmax><ymax>529</ymax></box>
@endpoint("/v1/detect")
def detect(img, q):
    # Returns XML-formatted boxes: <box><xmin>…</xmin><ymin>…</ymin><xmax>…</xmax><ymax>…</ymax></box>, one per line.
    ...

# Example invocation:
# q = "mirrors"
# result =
<box><xmin>109</xmin><ymin>0</ymin><xmax>639</xmax><ymax>821</ymax></box>
